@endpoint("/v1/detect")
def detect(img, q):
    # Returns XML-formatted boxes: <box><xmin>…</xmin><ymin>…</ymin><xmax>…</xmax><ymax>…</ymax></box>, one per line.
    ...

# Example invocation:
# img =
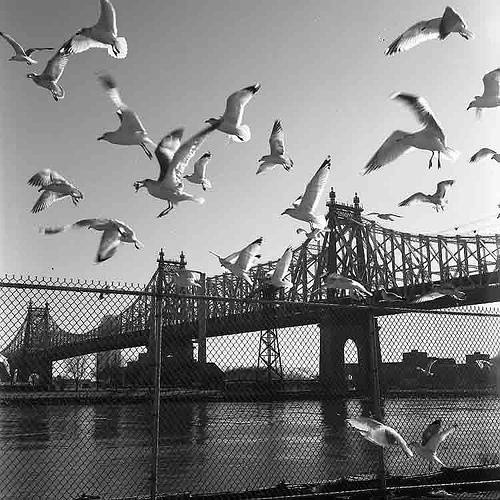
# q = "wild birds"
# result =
<box><xmin>24</xmin><ymin>168</ymin><xmax>83</xmax><ymax>214</ymax></box>
<box><xmin>256</xmin><ymin>119</ymin><xmax>294</xmax><ymax>177</ymax></box>
<box><xmin>359</xmin><ymin>89</ymin><xmax>456</xmax><ymax>177</ymax></box>
<box><xmin>404</xmin><ymin>416</ymin><xmax>458</xmax><ymax>469</ymax></box>
<box><xmin>396</xmin><ymin>179</ymin><xmax>455</xmax><ymax>213</ymax></box>
<box><xmin>345</xmin><ymin>414</ymin><xmax>415</xmax><ymax>462</ymax></box>
<box><xmin>280</xmin><ymin>157</ymin><xmax>331</xmax><ymax>227</ymax></box>
<box><xmin>38</xmin><ymin>216</ymin><xmax>140</xmax><ymax>265</ymax></box>
<box><xmin>1</xmin><ymin>0</ymin><xmax>131</xmax><ymax>103</ymax></box>
<box><xmin>470</xmin><ymin>146</ymin><xmax>500</xmax><ymax>165</ymax></box>
<box><xmin>382</xmin><ymin>6</ymin><xmax>472</xmax><ymax>58</ymax></box>
<box><xmin>95</xmin><ymin>76</ymin><xmax>261</xmax><ymax>220</ymax></box>
<box><xmin>168</xmin><ymin>229</ymin><xmax>468</xmax><ymax>305</ymax></box>
<box><xmin>466</xmin><ymin>67</ymin><xmax>500</xmax><ymax>112</ymax></box>
